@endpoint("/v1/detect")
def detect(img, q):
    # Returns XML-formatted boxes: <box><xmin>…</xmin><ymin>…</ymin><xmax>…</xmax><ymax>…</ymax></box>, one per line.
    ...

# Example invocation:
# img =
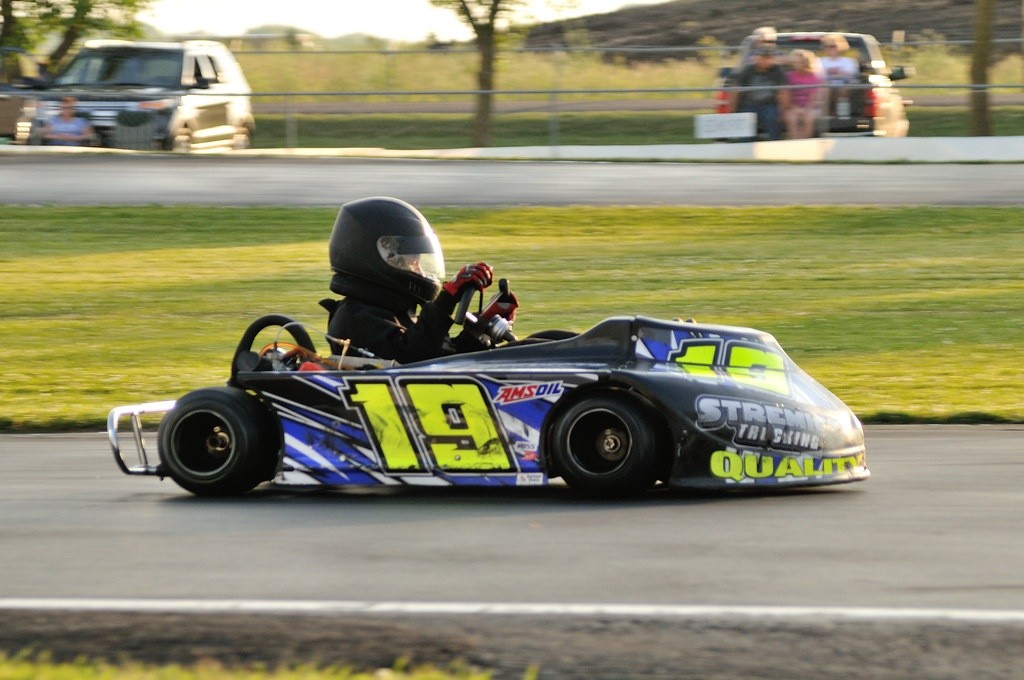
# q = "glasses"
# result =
<box><xmin>825</xmin><ymin>45</ymin><xmax>837</xmax><ymax>48</ymax></box>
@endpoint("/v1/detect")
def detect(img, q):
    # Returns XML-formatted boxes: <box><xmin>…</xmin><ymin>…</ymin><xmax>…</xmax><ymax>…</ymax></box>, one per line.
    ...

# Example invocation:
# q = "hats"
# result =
<box><xmin>326</xmin><ymin>194</ymin><xmax>446</xmax><ymax>315</ymax></box>
<box><xmin>748</xmin><ymin>46</ymin><xmax>784</xmax><ymax>55</ymax></box>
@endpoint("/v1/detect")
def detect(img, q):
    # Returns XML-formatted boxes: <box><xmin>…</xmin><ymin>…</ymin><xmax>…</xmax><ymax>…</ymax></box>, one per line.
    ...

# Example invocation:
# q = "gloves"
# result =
<box><xmin>444</xmin><ymin>260</ymin><xmax>495</xmax><ymax>296</ymax></box>
<box><xmin>481</xmin><ymin>290</ymin><xmax>520</xmax><ymax>321</ymax></box>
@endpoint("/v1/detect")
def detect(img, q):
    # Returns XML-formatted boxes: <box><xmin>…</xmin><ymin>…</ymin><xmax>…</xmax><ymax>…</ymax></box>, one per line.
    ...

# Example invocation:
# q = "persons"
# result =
<box><xmin>730</xmin><ymin>27</ymin><xmax>858</xmax><ymax>144</ymax></box>
<box><xmin>326</xmin><ymin>196</ymin><xmax>519</xmax><ymax>364</ymax></box>
<box><xmin>43</xmin><ymin>96</ymin><xmax>95</xmax><ymax>147</ymax></box>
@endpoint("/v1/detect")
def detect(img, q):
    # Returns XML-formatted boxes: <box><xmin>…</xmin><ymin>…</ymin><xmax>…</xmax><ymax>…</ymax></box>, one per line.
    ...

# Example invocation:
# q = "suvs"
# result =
<box><xmin>14</xmin><ymin>40</ymin><xmax>254</xmax><ymax>152</ymax></box>
<box><xmin>713</xmin><ymin>28</ymin><xmax>911</xmax><ymax>140</ymax></box>
